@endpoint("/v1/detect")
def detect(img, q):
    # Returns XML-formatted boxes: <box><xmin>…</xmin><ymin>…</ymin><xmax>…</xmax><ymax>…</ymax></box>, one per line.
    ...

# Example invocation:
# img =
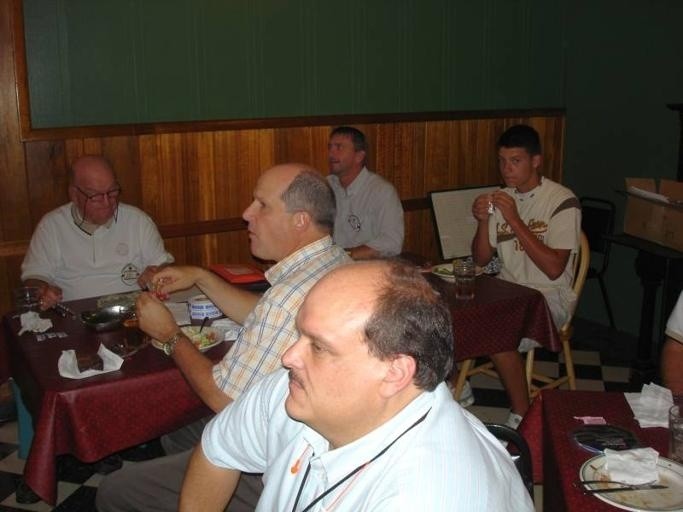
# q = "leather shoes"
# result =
<box><xmin>94</xmin><ymin>443</ymin><xmax>156</xmax><ymax>473</ymax></box>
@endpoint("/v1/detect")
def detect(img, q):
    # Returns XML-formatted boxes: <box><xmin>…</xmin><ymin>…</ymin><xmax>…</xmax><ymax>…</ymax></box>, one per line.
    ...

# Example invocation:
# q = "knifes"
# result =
<box><xmin>584</xmin><ymin>485</ymin><xmax>669</xmax><ymax>495</ymax></box>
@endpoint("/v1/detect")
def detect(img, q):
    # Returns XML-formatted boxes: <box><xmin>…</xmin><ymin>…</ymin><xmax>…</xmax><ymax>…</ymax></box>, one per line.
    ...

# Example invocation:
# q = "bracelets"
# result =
<box><xmin>343</xmin><ymin>247</ymin><xmax>352</xmax><ymax>257</ymax></box>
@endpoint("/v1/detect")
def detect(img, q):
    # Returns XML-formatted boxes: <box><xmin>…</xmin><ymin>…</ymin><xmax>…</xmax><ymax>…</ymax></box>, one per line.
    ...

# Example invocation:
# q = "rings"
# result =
<box><xmin>38</xmin><ymin>299</ymin><xmax>44</xmax><ymax>305</ymax></box>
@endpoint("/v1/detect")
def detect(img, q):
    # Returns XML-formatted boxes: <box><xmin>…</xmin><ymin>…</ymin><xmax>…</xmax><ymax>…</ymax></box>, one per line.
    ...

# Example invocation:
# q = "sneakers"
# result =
<box><xmin>498</xmin><ymin>410</ymin><xmax>523</xmax><ymax>449</ymax></box>
<box><xmin>446</xmin><ymin>378</ymin><xmax>475</xmax><ymax>408</ymax></box>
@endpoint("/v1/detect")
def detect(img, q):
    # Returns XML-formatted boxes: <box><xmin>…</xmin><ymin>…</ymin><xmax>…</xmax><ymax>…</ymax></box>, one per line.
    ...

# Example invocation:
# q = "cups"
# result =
<box><xmin>669</xmin><ymin>404</ymin><xmax>683</xmax><ymax>459</ymax></box>
<box><xmin>455</xmin><ymin>261</ymin><xmax>474</xmax><ymax>297</ymax></box>
<box><xmin>14</xmin><ymin>289</ymin><xmax>39</xmax><ymax>314</ymax></box>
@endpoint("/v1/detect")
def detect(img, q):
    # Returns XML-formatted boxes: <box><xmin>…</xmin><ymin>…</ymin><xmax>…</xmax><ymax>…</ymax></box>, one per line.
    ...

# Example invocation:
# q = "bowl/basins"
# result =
<box><xmin>81</xmin><ymin>305</ymin><xmax>136</xmax><ymax>331</ymax></box>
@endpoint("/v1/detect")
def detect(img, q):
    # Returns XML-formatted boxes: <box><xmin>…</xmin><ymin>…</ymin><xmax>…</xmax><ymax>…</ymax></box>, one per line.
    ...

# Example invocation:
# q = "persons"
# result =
<box><xmin>177</xmin><ymin>259</ymin><xmax>537</xmax><ymax>512</ymax></box>
<box><xmin>443</xmin><ymin>122</ymin><xmax>581</xmax><ymax>447</ymax></box>
<box><xmin>94</xmin><ymin>163</ymin><xmax>356</xmax><ymax>510</ymax></box>
<box><xmin>660</xmin><ymin>294</ymin><xmax>683</xmax><ymax>398</ymax></box>
<box><xmin>16</xmin><ymin>156</ymin><xmax>176</xmax><ymax>505</ymax></box>
<box><xmin>322</xmin><ymin>126</ymin><xmax>405</xmax><ymax>260</ymax></box>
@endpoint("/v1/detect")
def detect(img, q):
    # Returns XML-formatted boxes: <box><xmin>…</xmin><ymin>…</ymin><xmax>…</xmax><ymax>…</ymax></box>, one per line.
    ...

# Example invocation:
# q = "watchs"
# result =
<box><xmin>161</xmin><ymin>332</ymin><xmax>184</xmax><ymax>356</ymax></box>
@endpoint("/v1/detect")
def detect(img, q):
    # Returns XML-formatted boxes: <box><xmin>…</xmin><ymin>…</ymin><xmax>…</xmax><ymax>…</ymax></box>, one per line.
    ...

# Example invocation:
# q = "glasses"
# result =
<box><xmin>75</xmin><ymin>183</ymin><xmax>121</xmax><ymax>203</ymax></box>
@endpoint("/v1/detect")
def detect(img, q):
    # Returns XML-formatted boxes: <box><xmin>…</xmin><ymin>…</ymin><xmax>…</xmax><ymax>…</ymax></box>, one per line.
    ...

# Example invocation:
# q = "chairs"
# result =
<box><xmin>483</xmin><ymin>423</ymin><xmax>534</xmax><ymax>504</ymax></box>
<box><xmin>453</xmin><ymin>230</ymin><xmax>590</xmax><ymax>406</ymax></box>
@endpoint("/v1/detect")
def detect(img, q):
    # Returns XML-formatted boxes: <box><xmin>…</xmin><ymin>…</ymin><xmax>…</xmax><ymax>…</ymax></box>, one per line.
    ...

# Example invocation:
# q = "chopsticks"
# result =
<box><xmin>51</xmin><ymin>302</ymin><xmax>75</xmax><ymax>318</ymax></box>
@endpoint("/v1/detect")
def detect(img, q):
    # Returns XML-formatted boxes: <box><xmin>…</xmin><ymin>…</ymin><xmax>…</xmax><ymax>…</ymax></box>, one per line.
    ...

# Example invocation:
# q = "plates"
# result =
<box><xmin>434</xmin><ymin>263</ymin><xmax>482</xmax><ymax>282</ymax></box>
<box><xmin>580</xmin><ymin>453</ymin><xmax>683</xmax><ymax>512</ymax></box>
<box><xmin>152</xmin><ymin>326</ymin><xmax>222</xmax><ymax>357</ymax></box>
